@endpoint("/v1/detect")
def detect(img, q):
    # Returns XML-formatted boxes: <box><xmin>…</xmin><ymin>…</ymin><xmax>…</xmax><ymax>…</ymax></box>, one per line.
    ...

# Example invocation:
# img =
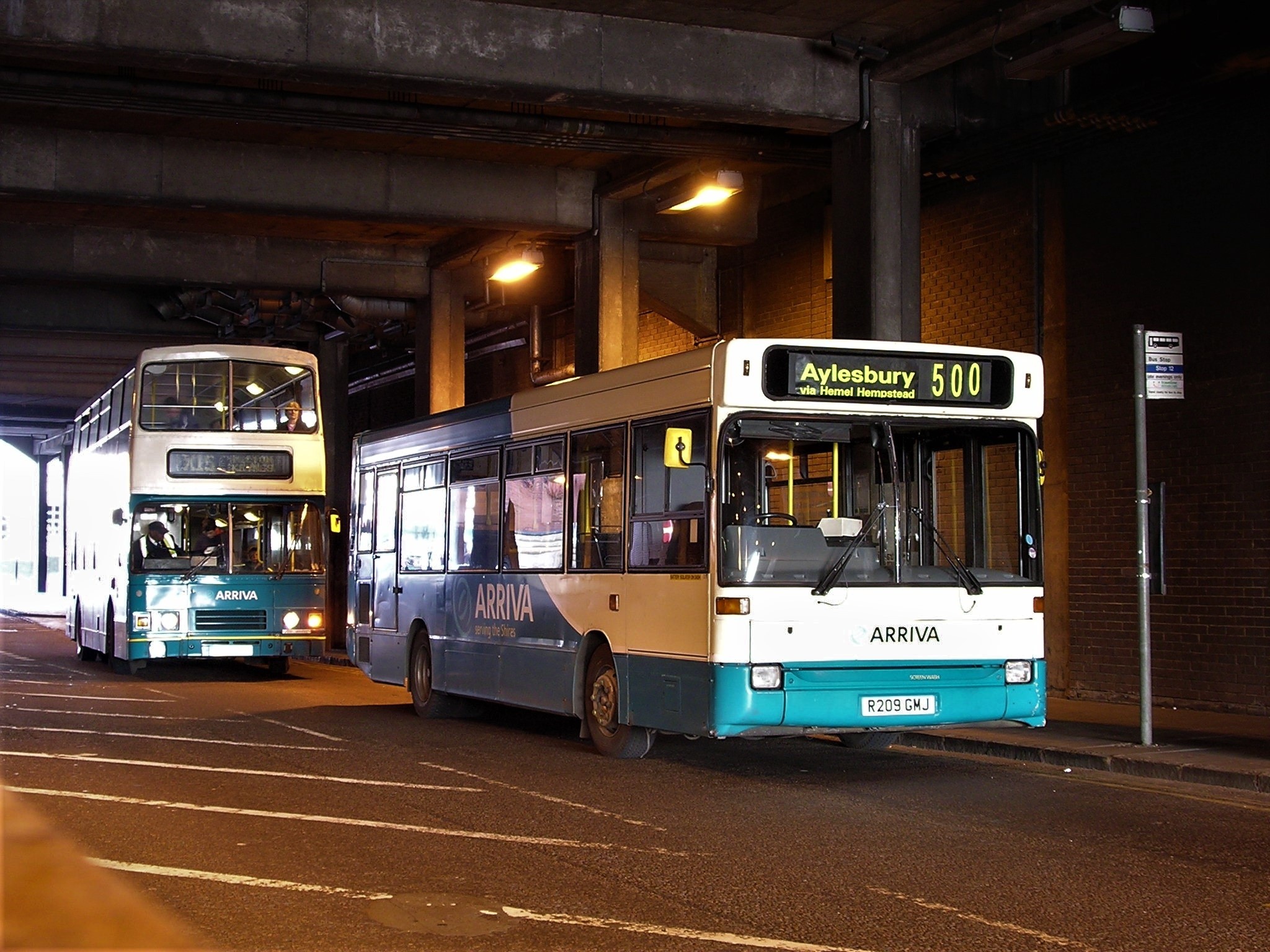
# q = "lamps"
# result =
<box><xmin>486</xmin><ymin>249</ymin><xmax>545</xmax><ymax>281</ymax></box>
<box><xmin>656</xmin><ymin>170</ymin><xmax>745</xmax><ymax>215</ymax></box>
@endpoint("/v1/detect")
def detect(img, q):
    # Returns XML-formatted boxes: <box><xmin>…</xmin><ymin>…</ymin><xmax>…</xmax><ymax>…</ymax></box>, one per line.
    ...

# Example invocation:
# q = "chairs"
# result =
<box><xmin>458</xmin><ymin>524</ymin><xmax>622</xmax><ymax>569</ymax></box>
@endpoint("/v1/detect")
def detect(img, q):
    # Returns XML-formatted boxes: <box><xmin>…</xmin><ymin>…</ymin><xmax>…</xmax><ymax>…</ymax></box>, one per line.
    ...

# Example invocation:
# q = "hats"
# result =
<box><xmin>148</xmin><ymin>521</ymin><xmax>169</xmax><ymax>534</ymax></box>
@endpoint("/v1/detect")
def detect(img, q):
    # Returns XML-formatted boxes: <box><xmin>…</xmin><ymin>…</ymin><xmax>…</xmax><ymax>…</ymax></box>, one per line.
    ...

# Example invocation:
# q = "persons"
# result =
<box><xmin>157</xmin><ymin>396</ymin><xmax>202</xmax><ymax>431</ymax></box>
<box><xmin>242</xmin><ymin>546</ymin><xmax>269</xmax><ymax>572</ymax></box>
<box><xmin>213</xmin><ymin>411</ymin><xmax>237</xmax><ymax>431</ymax></box>
<box><xmin>276</xmin><ymin>402</ymin><xmax>312</xmax><ymax>433</ymax></box>
<box><xmin>190</xmin><ymin>520</ymin><xmax>237</xmax><ymax>569</ymax></box>
<box><xmin>133</xmin><ymin>522</ymin><xmax>187</xmax><ymax>573</ymax></box>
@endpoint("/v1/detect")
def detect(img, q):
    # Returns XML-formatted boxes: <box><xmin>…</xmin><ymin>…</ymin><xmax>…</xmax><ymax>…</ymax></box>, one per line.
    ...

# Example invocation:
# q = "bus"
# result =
<box><xmin>64</xmin><ymin>344</ymin><xmax>341</xmax><ymax>673</ymax></box>
<box><xmin>346</xmin><ymin>337</ymin><xmax>1048</xmax><ymax>758</ymax></box>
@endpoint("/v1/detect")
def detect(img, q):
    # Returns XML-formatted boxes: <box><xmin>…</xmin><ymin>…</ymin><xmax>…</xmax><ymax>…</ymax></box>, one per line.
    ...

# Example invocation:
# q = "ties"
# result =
<box><xmin>155</xmin><ymin>540</ymin><xmax>161</xmax><ymax>548</ymax></box>
